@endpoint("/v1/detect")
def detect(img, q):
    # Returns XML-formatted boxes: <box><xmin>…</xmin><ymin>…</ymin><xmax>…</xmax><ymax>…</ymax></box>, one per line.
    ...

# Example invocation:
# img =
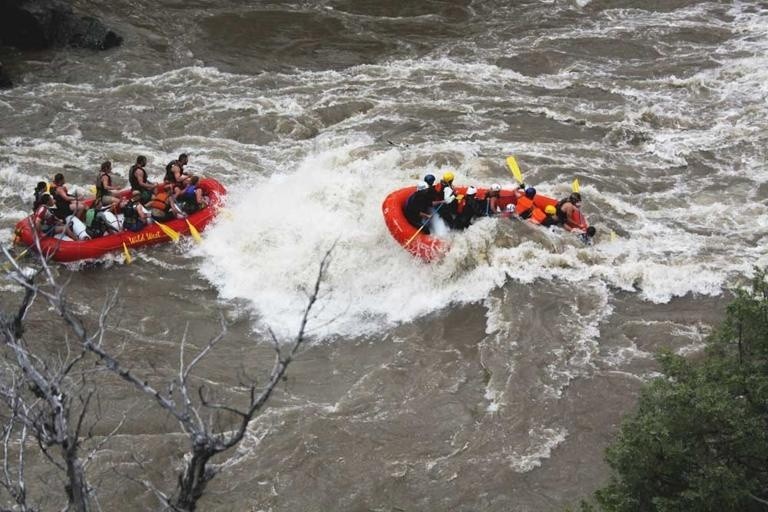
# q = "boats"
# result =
<box><xmin>381</xmin><ymin>184</ymin><xmax>588</xmax><ymax>262</ymax></box>
<box><xmin>14</xmin><ymin>179</ymin><xmax>228</xmax><ymax>262</ymax></box>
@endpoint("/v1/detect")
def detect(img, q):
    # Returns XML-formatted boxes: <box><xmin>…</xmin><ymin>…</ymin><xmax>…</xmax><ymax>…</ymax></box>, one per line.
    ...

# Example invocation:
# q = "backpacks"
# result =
<box><xmin>85</xmin><ymin>208</ymin><xmax>110</xmax><ymax>228</ymax></box>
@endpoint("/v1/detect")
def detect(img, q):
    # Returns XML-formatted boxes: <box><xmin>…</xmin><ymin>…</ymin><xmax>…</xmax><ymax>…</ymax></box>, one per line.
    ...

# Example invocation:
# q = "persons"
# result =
<box><xmin>32</xmin><ymin>153</ymin><xmax>210</xmax><ymax>241</ymax></box>
<box><xmin>406</xmin><ymin>173</ymin><xmax>595</xmax><ymax>246</ymax></box>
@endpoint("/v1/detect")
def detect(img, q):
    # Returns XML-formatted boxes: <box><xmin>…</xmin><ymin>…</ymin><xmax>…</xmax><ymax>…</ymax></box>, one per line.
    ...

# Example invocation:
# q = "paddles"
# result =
<box><xmin>174</xmin><ymin>198</ymin><xmax>200</xmax><ymax>241</ymax></box>
<box><xmin>150</xmin><ymin>217</ymin><xmax>179</xmax><ymax>240</ymax></box>
<box><xmin>3</xmin><ymin>223</ymin><xmax>59</xmax><ymax>270</ymax></box>
<box><xmin>506</xmin><ymin>156</ymin><xmax>525</xmax><ymax>189</ymax></box>
<box><xmin>113</xmin><ymin>205</ymin><xmax>132</xmax><ymax>264</ymax></box>
<box><xmin>572</xmin><ymin>179</ymin><xmax>579</xmax><ymax>193</ymax></box>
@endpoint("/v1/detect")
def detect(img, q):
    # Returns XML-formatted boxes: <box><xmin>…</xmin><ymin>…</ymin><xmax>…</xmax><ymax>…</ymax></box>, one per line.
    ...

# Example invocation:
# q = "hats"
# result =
<box><xmin>102</xmin><ymin>195</ymin><xmax>112</xmax><ymax>204</ymax></box>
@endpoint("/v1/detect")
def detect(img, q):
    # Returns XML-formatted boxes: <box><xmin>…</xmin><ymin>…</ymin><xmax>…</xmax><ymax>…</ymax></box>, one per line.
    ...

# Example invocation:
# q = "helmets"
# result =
<box><xmin>442</xmin><ymin>172</ymin><xmax>455</xmax><ymax>182</ymax></box>
<box><xmin>417</xmin><ymin>181</ymin><xmax>429</xmax><ymax>191</ymax></box>
<box><xmin>506</xmin><ymin>204</ymin><xmax>516</xmax><ymax>213</ymax></box>
<box><xmin>569</xmin><ymin>192</ymin><xmax>583</xmax><ymax>202</ymax></box>
<box><xmin>466</xmin><ymin>185</ymin><xmax>477</xmax><ymax>195</ymax></box>
<box><xmin>544</xmin><ymin>205</ymin><xmax>557</xmax><ymax>215</ymax></box>
<box><xmin>525</xmin><ymin>188</ymin><xmax>536</xmax><ymax>198</ymax></box>
<box><xmin>424</xmin><ymin>174</ymin><xmax>436</xmax><ymax>183</ymax></box>
<box><xmin>490</xmin><ymin>183</ymin><xmax>501</xmax><ymax>192</ymax></box>
<box><xmin>586</xmin><ymin>226</ymin><xmax>596</xmax><ymax>237</ymax></box>
